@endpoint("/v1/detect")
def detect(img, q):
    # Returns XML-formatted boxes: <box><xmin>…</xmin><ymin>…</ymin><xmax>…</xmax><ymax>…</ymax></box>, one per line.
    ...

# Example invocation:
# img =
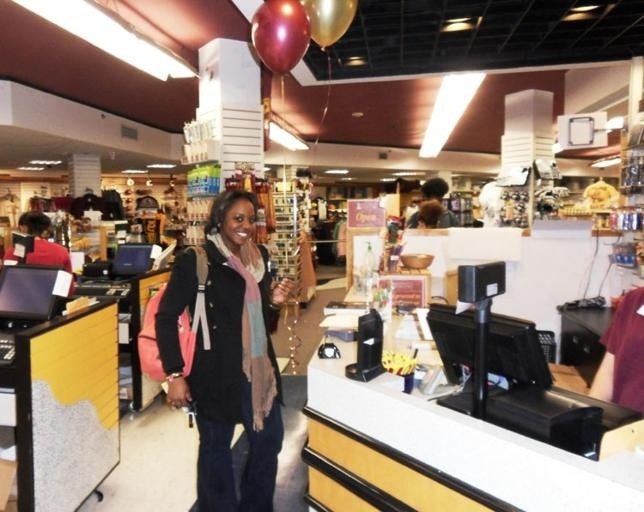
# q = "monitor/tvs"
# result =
<box><xmin>0</xmin><ymin>265</ymin><xmax>64</xmax><ymax>322</ymax></box>
<box><xmin>426</xmin><ymin>303</ymin><xmax>553</xmax><ymax>391</ymax></box>
<box><xmin>114</xmin><ymin>243</ymin><xmax>154</xmax><ymax>276</ymax></box>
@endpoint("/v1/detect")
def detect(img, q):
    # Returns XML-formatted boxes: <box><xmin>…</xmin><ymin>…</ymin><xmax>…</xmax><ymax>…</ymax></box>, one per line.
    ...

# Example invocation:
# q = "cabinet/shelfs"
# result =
<box><xmin>179</xmin><ymin>155</ymin><xmax>220</xmax><ymax>249</ymax></box>
<box><xmin>559</xmin><ymin>306</ymin><xmax>616</xmax><ymax>385</ymax></box>
<box><xmin>612</xmin><ymin>143</ymin><xmax>644</xmax><ymax>290</ymax></box>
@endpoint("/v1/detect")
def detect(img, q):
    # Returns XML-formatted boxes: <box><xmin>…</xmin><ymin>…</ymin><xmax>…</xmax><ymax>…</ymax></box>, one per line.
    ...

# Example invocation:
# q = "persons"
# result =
<box><xmin>153</xmin><ymin>187</ymin><xmax>294</xmax><ymax>512</ymax></box>
<box><xmin>406</xmin><ymin>177</ymin><xmax>460</xmax><ymax>229</ymax></box>
<box><xmin>416</xmin><ymin>198</ymin><xmax>443</xmax><ymax>229</ymax></box>
<box><xmin>587</xmin><ymin>285</ymin><xmax>644</xmax><ymax>415</ymax></box>
<box><xmin>3</xmin><ymin>211</ymin><xmax>74</xmax><ymax>299</ymax></box>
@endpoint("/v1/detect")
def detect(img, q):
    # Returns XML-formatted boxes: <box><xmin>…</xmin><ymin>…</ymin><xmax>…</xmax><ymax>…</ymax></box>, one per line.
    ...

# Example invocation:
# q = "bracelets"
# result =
<box><xmin>170</xmin><ymin>372</ymin><xmax>183</xmax><ymax>378</ymax></box>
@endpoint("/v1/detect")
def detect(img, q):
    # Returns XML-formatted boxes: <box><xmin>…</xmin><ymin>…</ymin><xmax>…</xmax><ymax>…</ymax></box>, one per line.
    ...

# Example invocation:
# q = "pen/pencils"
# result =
<box><xmin>407</xmin><ymin>348</ymin><xmax>419</xmax><ymax>375</ymax></box>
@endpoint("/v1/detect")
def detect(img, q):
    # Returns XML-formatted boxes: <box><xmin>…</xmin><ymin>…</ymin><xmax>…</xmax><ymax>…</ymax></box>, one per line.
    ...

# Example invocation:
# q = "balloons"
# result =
<box><xmin>251</xmin><ymin>0</ymin><xmax>312</xmax><ymax>77</ymax></box>
<box><xmin>300</xmin><ymin>0</ymin><xmax>357</xmax><ymax>51</ymax></box>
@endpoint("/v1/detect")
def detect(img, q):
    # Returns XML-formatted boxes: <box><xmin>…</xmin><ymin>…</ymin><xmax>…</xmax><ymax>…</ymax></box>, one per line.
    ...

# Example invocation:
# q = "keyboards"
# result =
<box><xmin>0</xmin><ymin>337</ymin><xmax>16</xmax><ymax>364</ymax></box>
<box><xmin>73</xmin><ymin>284</ymin><xmax>130</xmax><ymax>298</ymax></box>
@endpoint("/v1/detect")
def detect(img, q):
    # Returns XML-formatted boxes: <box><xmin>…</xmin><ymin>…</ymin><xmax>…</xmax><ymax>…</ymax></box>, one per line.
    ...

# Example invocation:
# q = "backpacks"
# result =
<box><xmin>138</xmin><ymin>247</ymin><xmax>210</xmax><ymax>381</ymax></box>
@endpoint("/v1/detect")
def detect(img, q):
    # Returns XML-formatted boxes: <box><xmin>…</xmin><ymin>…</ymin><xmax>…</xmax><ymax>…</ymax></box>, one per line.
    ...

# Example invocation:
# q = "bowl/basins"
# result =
<box><xmin>400</xmin><ymin>254</ymin><xmax>435</xmax><ymax>269</ymax></box>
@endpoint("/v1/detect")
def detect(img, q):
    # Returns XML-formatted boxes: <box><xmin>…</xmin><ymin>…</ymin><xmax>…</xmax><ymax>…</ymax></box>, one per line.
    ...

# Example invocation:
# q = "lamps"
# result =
<box><xmin>11</xmin><ymin>0</ymin><xmax>203</xmax><ymax>83</ymax></box>
<box><xmin>126</xmin><ymin>173</ymin><xmax>136</xmax><ymax>186</ymax></box>
<box><xmin>145</xmin><ymin>170</ymin><xmax>154</xmax><ymax>187</ymax></box>
<box><xmin>266</xmin><ymin>119</ymin><xmax>311</xmax><ymax>152</ymax></box>
<box><xmin>168</xmin><ymin>172</ymin><xmax>176</xmax><ymax>188</ymax></box>
